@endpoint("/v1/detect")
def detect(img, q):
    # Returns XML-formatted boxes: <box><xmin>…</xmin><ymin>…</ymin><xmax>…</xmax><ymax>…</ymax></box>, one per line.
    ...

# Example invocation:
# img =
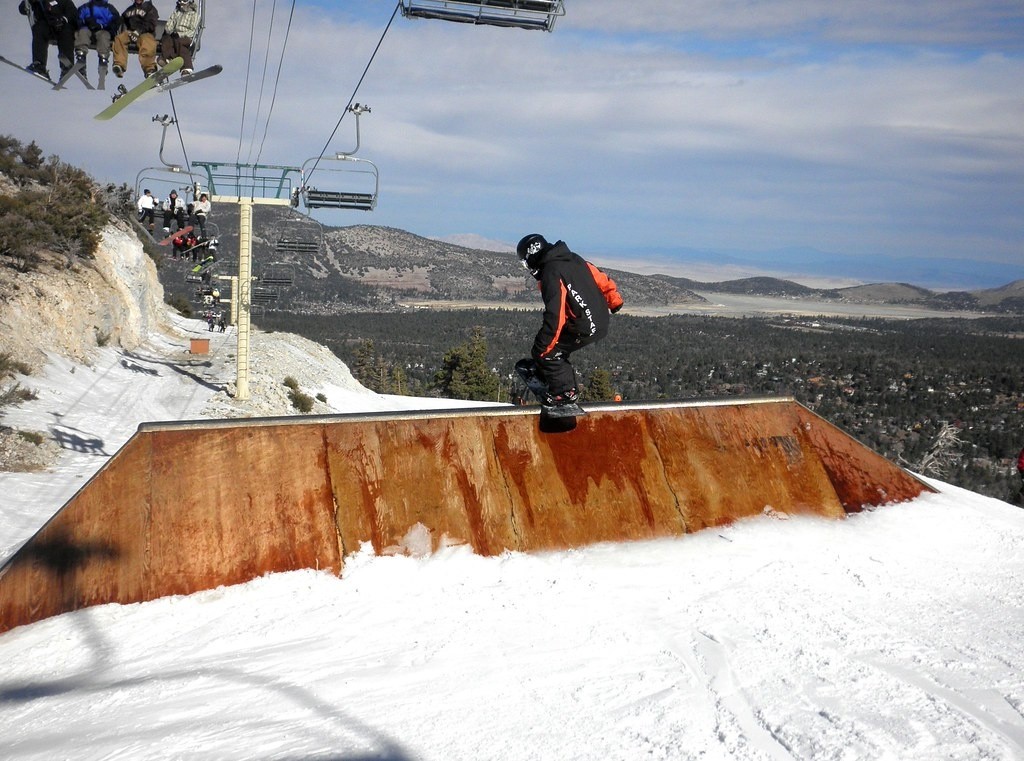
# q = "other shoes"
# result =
<box><xmin>150</xmin><ymin>223</ymin><xmax>154</xmax><ymax>230</ymax></box>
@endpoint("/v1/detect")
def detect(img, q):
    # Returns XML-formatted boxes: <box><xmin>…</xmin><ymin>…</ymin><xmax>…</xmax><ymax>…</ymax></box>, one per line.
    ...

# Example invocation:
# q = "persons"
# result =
<box><xmin>137</xmin><ymin>189</ymin><xmax>227</xmax><ymax>333</ymax></box>
<box><xmin>1018</xmin><ymin>447</ymin><xmax>1024</xmax><ymax>485</ymax></box>
<box><xmin>517</xmin><ymin>234</ymin><xmax>624</xmax><ymax>407</ymax></box>
<box><xmin>19</xmin><ymin>0</ymin><xmax>199</xmax><ymax>81</ymax></box>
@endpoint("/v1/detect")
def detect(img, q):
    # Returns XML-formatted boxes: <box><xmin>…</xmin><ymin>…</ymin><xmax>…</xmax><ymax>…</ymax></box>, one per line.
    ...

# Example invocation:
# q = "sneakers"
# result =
<box><xmin>180</xmin><ymin>68</ymin><xmax>193</xmax><ymax>75</ymax></box>
<box><xmin>162</xmin><ymin>227</ymin><xmax>170</xmax><ymax>233</ymax></box>
<box><xmin>156</xmin><ymin>57</ymin><xmax>170</xmax><ymax>68</ymax></box>
<box><xmin>26</xmin><ymin>61</ymin><xmax>47</xmax><ymax>73</ymax></box>
<box><xmin>74</xmin><ymin>50</ymin><xmax>85</xmax><ymax>62</ymax></box>
<box><xmin>542</xmin><ymin>388</ymin><xmax>580</xmax><ymax>406</ymax></box>
<box><xmin>99</xmin><ymin>58</ymin><xmax>108</xmax><ymax>66</ymax></box>
<box><xmin>516</xmin><ymin>358</ymin><xmax>539</xmax><ymax>375</ymax></box>
<box><xmin>145</xmin><ymin>72</ymin><xmax>153</xmax><ymax>77</ymax></box>
<box><xmin>176</xmin><ymin>228</ymin><xmax>183</xmax><ymax>232</ymax></box>
<box><xmin>112</xmin><ymin>65</ymin><xmax>124</xmax><ymax>79</ymax></box>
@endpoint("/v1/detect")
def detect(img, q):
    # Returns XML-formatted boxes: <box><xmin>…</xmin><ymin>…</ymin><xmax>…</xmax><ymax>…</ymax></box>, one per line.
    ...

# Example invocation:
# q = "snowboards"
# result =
<box><xmin>514</xmin><ymin>356</ymin><xmax>589</xmax><ymax>421</ymax></box>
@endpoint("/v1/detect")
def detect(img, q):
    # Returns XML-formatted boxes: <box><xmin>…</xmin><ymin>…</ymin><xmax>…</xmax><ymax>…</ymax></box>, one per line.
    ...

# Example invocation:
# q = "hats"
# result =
<box><xmin>144</xmin><ymin>189</ymin><xmax>149</xmax><ymax>193</ymax></box>
<box><xmin>171</xmin><ymin>190</ymin><xmax>177</xmax><ymax>195</ymax></box>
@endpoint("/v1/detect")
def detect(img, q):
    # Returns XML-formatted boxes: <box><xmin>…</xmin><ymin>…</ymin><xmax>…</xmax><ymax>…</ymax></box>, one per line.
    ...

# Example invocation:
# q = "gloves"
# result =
<box><xmin>89</xmin><ymin>23</ymin><xmax>104</xmax><ymax>31</ymax></box>
<box><xmin>170</xmin><ymin>31</ymin><xmax>179</xmax><ymax>39</ymax></box>
<box><xmin>53</xmin><ymin>16</ymin><xmax>69</xmax><ymax>29</ymax></box>
<box><xmin>129</xmin><ymin>29</ymin><xmax>140</xmax><ymax>42</ymax></box>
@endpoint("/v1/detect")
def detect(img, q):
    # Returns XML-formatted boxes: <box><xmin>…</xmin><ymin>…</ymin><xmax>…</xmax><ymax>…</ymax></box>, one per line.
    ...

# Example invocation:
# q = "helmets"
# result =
<box><xmin>516</xmin><ymin>233</ymin><xmax>553</xmax><ymax>279</ymax></box>
